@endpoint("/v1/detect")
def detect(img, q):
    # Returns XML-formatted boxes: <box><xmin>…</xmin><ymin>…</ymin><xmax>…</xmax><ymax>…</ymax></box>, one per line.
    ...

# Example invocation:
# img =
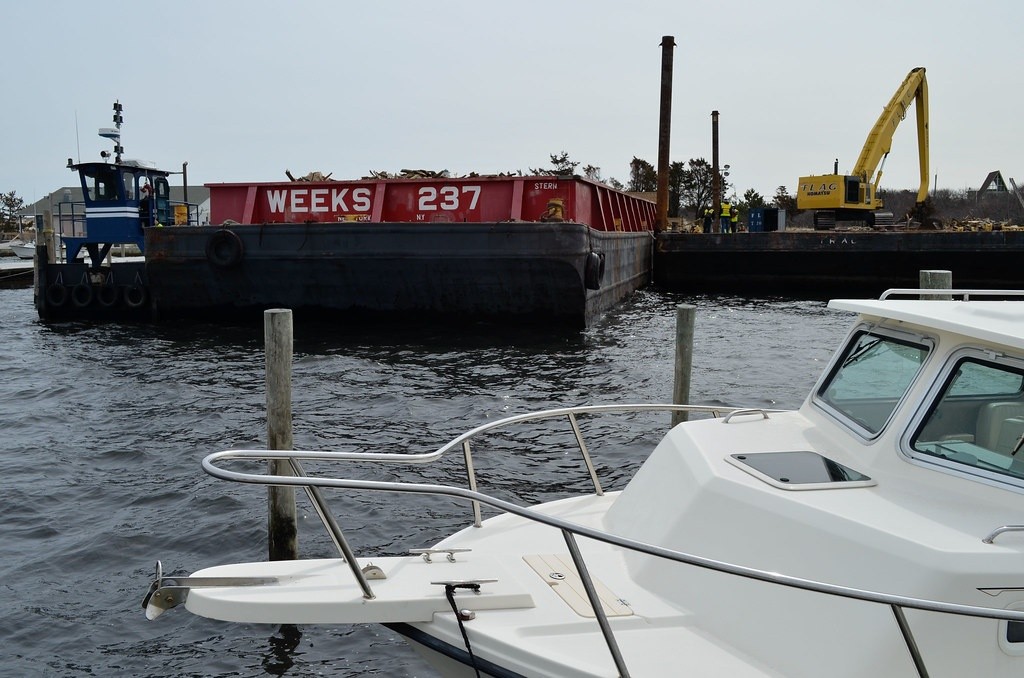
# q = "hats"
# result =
<box><xmin>707</xmin><ymin>204</ymin><xmax>712</xmax><ymax>208</ymax></box>
<box><xmin>724</xmin><ymin>197</ymin><xmax>728</xmax><ymax>200</ymax></box>
<box><xmin>732</xmin><ymin>204</ymin><xmax>735</xmax><ymax>207</ymax></box>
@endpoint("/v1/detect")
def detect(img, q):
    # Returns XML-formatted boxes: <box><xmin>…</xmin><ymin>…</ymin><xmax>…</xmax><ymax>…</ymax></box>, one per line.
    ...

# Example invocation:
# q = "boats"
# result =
<box><xmin>139</xmin><ymin>286</ymin><xmax>1024</xmax><ymax>678</ymax></box>
<box><xmin>8</xmin><ymin>232</ymin><xmax>91</xmax><ymax>260</ymax></box>
<box><xmin>27</xmin><ymin>97</ymin><xmax>203</xmax><ymax>329</ymax></box>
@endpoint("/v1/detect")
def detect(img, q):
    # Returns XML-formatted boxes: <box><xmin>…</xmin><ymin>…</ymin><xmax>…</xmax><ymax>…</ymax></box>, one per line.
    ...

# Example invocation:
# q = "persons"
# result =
<box><xmin>720</xmin><ymin>196</ymin><xmax>739</xmax><ymax>233</ymax></box>
<box><xmin>126</xmin><ymin>190</ymin><xmax>132</xmax><ymax>199</ymax></box>
<box><xmin>702</xmin><ymin>204</ymin><xmax>716</xmax><ymax>233</ymax></box>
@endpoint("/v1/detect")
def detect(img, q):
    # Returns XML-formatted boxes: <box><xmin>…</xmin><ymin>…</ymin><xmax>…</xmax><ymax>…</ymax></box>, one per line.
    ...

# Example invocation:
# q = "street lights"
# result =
<box><xmin>719</xmin><ymin>164</ymin><xmax>731</xmax><ymax>204</ymax></box>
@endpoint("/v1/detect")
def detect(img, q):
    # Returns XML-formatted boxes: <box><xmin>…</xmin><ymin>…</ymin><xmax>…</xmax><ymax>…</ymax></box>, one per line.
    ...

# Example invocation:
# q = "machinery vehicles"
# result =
<box><xmin>793</xmin><ymin>64</ymin><xmax>947</xmax><ymax>231</ymax></box>
